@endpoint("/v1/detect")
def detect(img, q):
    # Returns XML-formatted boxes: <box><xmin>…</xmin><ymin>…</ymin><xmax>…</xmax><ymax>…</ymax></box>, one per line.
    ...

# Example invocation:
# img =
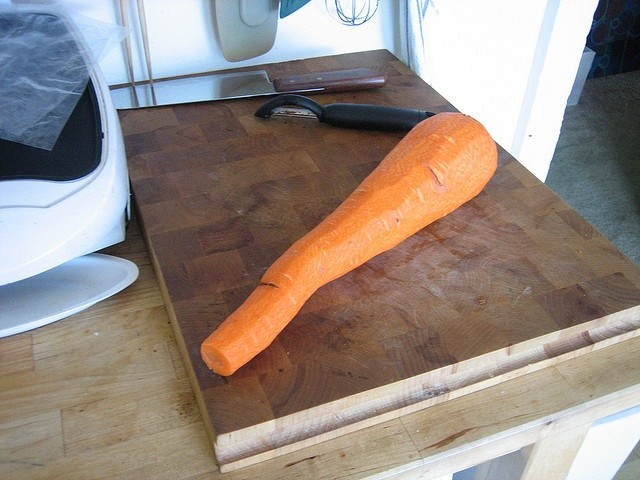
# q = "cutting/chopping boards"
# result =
<box><xmin>108</xmin><ymin>46</ymin><xmax>640</xmax><ymax>474</ymax></box>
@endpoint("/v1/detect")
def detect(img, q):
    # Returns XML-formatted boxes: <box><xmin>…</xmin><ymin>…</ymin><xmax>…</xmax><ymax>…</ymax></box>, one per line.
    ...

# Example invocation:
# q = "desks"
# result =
<box><xmin>0</xmin><ymin>222</ymin><xmax>640</xmax><ymax>479</ymax></box>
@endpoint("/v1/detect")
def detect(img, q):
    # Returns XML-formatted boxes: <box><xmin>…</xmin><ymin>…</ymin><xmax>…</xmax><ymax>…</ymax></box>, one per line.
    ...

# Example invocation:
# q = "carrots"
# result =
<box><xmin>200</xmin><ymin>111</ymin><xmax>497</xmax><ymax>376</ymax></box>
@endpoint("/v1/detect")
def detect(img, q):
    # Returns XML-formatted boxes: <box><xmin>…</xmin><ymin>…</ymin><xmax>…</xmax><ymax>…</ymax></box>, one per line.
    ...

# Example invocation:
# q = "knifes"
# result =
<box><xmin>109</xmin><ymin>68</ymin><xmax>387</xmax><ymax>110</ymax></box>
